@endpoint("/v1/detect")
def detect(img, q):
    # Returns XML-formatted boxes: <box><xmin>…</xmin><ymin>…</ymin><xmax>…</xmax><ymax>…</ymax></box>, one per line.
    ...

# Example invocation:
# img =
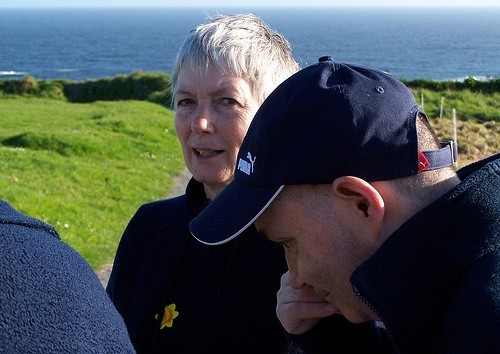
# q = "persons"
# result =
<box><xmin>188</xmin><ymin>55</ymin><xmax>500</xmax><ymax>354</ymax></box>
<box><xmin>105</xmin><ymin>13</ymin><xmax>304</xmax><ymax>354</ymax></box>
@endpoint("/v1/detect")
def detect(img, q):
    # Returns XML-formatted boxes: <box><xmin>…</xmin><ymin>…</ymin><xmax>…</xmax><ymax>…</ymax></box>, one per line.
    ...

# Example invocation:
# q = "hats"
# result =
<box><xmin>189</xmin><ymin>56</ymin><xmax>457</xmax><ymax>246</ymax></box>
